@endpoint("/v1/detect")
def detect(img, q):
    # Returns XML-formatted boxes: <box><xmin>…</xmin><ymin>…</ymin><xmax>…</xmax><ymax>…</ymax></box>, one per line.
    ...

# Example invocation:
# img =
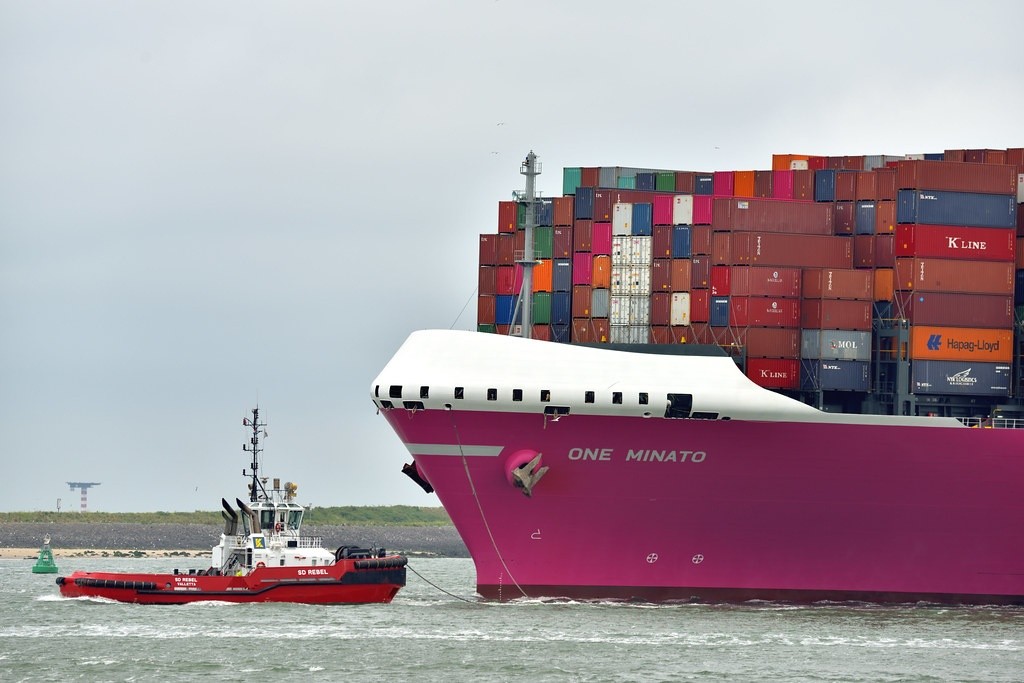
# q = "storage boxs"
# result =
<box><xmin>476</xmin><ymin>148</ymin><xmax>1024</xmax><ymax>427</ymax></box>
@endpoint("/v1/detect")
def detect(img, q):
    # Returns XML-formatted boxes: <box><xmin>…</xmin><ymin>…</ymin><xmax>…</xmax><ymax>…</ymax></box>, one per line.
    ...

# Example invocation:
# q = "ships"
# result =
<box><xmin>370</xmin><ymin>148</ymin><xmax>1024</xmax><ymax>607</ymax></box>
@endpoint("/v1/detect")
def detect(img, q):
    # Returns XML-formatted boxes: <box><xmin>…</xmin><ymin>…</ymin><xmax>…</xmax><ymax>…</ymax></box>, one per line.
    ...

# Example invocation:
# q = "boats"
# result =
<box><xmin>55</xmin><ymin>404</ymin><xmax>409</xmax><ymax>607</ymax></box>
